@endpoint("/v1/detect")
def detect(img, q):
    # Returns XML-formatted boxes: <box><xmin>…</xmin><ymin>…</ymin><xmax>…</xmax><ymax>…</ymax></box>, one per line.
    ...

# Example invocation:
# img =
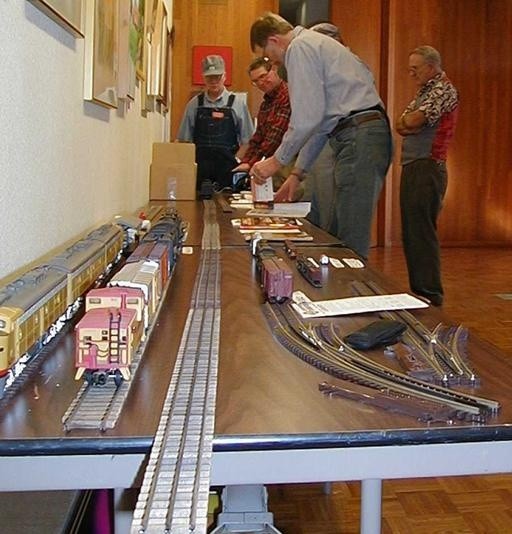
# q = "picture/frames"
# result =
<box><xmin>30</xmin><ymin>0</ymin><xmax>171</xmax><ymax>111</ymax></box>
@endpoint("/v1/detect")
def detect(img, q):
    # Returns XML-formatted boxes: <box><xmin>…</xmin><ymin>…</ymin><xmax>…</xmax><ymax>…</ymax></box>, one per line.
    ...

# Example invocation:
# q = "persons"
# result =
<box><xmin>247</xmin><ymin>14</ymin><xmax>393</xmax><ymax>262</ymax></box>
<box><xmin>176</xmin><ymin>54</ymin><xmax>254</xmax><ymax>190</ymax></box>
<box><xmin>395</xmin><ymin>45</ymin><xmax>459</xmax><ymax>306</ymax></box>
<box><xmin>301</xmin><ymin>22</ymin><xmax>362</xmax><ymax>231</ymax></box>
<box><xmin>232</xmin><ymin>57</ymin><xmax>306</xmax><ymax>203</ymax></box>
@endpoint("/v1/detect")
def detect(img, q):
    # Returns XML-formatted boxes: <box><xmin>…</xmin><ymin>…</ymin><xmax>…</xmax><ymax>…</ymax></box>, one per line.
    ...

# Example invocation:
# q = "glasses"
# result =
<box><xmin>250</xmin><ymin>67</ymin><xmax>271</xmax><ymax>86</ymax></box>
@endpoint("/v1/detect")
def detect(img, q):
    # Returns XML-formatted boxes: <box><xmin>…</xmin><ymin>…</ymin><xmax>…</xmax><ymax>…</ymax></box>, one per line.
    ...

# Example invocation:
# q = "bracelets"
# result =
<box><xmin>290</xmin><ymin>172</ymin><xmax>302</xmax><ymax>182</ymax></box>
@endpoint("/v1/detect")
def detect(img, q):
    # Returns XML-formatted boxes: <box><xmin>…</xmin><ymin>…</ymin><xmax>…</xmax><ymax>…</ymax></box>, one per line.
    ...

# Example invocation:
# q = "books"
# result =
<box><xmin>228</xmin><ymin>189</ymin><xmax>315</xmax><ymax>242</ymax></box>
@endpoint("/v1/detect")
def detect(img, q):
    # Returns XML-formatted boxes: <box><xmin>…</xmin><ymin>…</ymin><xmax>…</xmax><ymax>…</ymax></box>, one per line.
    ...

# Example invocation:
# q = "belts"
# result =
<box><xmin>327</xmin><ymin>110</ymin><xmax>381</xmax><ymax>137</ymax></box>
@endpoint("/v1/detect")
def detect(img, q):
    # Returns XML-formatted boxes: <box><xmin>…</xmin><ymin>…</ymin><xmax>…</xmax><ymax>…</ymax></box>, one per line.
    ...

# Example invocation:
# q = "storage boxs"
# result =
<box><xmin>148</xmin><ymin>141</ymin><xmax>198</xmax><ymax>200</ymax></box>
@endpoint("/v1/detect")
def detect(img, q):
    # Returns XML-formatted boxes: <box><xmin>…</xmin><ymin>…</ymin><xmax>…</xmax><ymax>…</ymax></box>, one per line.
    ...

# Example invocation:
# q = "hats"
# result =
<box><xmin>202</xmin><ymin>54</ymin><xmax>226</xmax><ymax>76</ymax></box>
